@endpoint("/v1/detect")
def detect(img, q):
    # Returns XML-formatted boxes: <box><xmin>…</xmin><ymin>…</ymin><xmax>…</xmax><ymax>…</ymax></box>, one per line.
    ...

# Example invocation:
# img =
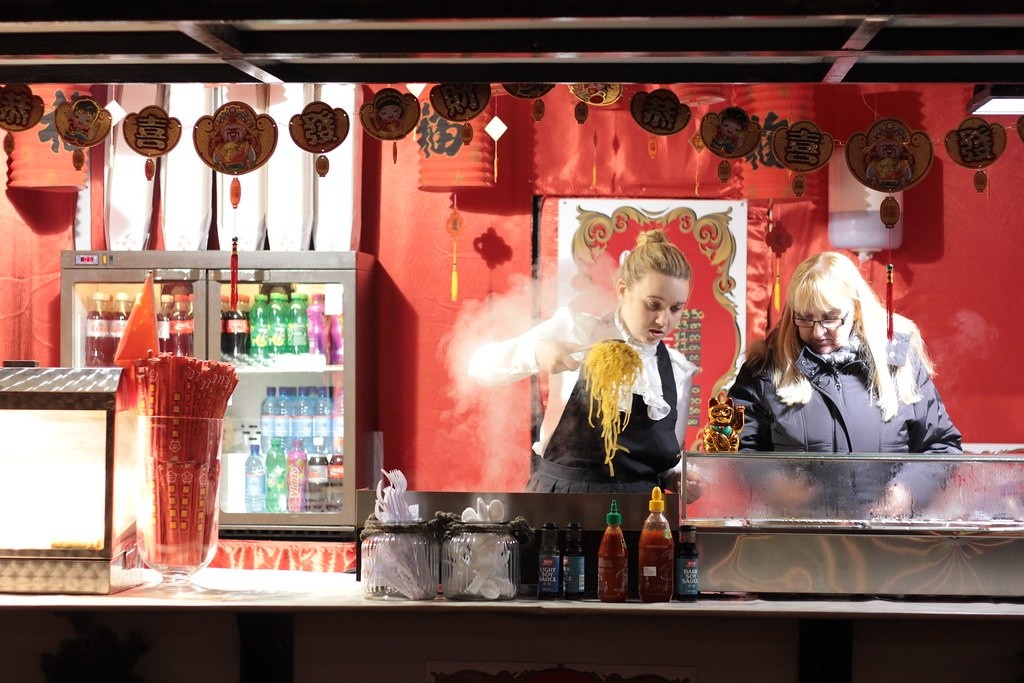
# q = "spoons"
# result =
<box><xmin>450</xmin><ymin>497</ymin><xmax>516</xmax><ymax>598</ymax></box>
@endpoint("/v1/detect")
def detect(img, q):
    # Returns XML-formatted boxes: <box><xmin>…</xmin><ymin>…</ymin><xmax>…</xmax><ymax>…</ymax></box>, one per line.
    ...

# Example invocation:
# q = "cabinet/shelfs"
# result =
<box><xmin>59</xmin><ymin>250</ymin><xmax>376</xmax><ymax>527</ymax></box>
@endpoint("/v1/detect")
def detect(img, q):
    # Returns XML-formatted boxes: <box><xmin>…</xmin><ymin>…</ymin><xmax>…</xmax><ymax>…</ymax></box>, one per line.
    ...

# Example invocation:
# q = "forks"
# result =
<box><xmin>373</xmin><ymin>467</ymin><xmax>424</xmax><ymax>599</ymax></box>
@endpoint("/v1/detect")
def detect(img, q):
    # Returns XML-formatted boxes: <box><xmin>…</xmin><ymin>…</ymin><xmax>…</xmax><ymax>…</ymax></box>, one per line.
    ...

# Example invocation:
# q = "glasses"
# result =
<box><xmin>790</xmin><ymin>310</ymin><xmax>850</xmax><ymax>331</ymax></box>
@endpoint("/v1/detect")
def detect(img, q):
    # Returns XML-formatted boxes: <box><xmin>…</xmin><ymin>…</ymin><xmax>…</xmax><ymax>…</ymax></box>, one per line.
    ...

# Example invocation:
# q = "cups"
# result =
<box><xmin>134</xmin><ymin>415</ymin><xmax>225</xmax><ymax>595</ymax></box>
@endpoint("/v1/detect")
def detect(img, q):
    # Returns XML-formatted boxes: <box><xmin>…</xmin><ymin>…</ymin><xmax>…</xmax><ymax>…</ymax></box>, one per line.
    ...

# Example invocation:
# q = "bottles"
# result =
<box><xmin>220</xmin><ymin>293</ymin><xmax>344</xmax><ymax>366</ymax></box>
<box><xmin>360</xmin><ymin>522</ymin><xmax>439</xmax><ymax>600</ymax></box>
<box><xmin>638</xmin><ymin>486</ymin><xmax>674</xmax><ymax>602</ymax></box>
<box><xmin>561</xmin><ymin>521</ymin><xmax>586</xmax><ymax>599</ymax></box>
<box><xmin>245</xmin><ymin>385</ymin><xmax>344</xmax><ymax>513</ymax></box>
<box><xmin>597</xmin><ymin>500</ymin><xmax>628</xmax><ymax>602</ymax></box>
<box><xmin>441</xmin><ymin>522</ymin><xmax>521</xmax><ymax>600</ymax></box>
<box><xmin>674</xmin><ymin>525</ymin><xmax>699</xmax><ymax>601</ymax></box>
<box><xmin>84</xmin><ymin>292</ymin><xmax>194</xmax><ymax>367</ymax></box>
<box><xmin>537</xmin><ymin>522</ymin><xmax>560</xmax><ymax>600</ymax></box>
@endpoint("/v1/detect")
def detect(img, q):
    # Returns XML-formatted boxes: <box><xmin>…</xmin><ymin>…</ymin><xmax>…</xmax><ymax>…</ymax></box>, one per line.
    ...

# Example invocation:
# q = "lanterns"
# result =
<box><xmin>6</xmin><ymin>82</ymin><xmax>88</xmax><ymax>193</ymax></box>
<box><xmin>416</xmin><ymin>83</ymin><xmax>493</xmax><ymax>296</ymax></box>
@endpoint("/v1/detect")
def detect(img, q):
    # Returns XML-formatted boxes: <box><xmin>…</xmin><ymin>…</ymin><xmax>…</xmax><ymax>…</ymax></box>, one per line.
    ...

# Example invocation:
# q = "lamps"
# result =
<box><xmin>966</xmin><ymin>84</ymin><xmax>1024</xmax><ymax>115</ymax></box>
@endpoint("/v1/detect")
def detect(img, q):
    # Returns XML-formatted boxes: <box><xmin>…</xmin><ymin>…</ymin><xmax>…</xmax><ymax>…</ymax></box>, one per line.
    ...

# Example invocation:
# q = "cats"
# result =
<box><xmin>702</xmin><ymin>397</ymin><xmax>745</xmax><ymax>453</ymax></box>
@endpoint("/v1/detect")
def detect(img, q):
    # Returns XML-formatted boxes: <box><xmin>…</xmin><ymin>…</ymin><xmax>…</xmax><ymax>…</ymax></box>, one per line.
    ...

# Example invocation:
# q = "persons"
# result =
<box><xmin>466</xmin><ymin>230</ymin><xmax>701</xmax><ymax>492</ymax></box>
<box><xmin>724</xmin><ymin>251</ymin><xmax>963</xmax><ymax>517</ymax></box>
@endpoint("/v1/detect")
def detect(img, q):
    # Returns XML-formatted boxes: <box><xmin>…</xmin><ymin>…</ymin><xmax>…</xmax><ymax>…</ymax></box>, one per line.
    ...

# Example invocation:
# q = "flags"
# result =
<box><xmin>114</xmin><ymin>275</ymin><xmax>161</xmax><ymax>366</ymax></box>
